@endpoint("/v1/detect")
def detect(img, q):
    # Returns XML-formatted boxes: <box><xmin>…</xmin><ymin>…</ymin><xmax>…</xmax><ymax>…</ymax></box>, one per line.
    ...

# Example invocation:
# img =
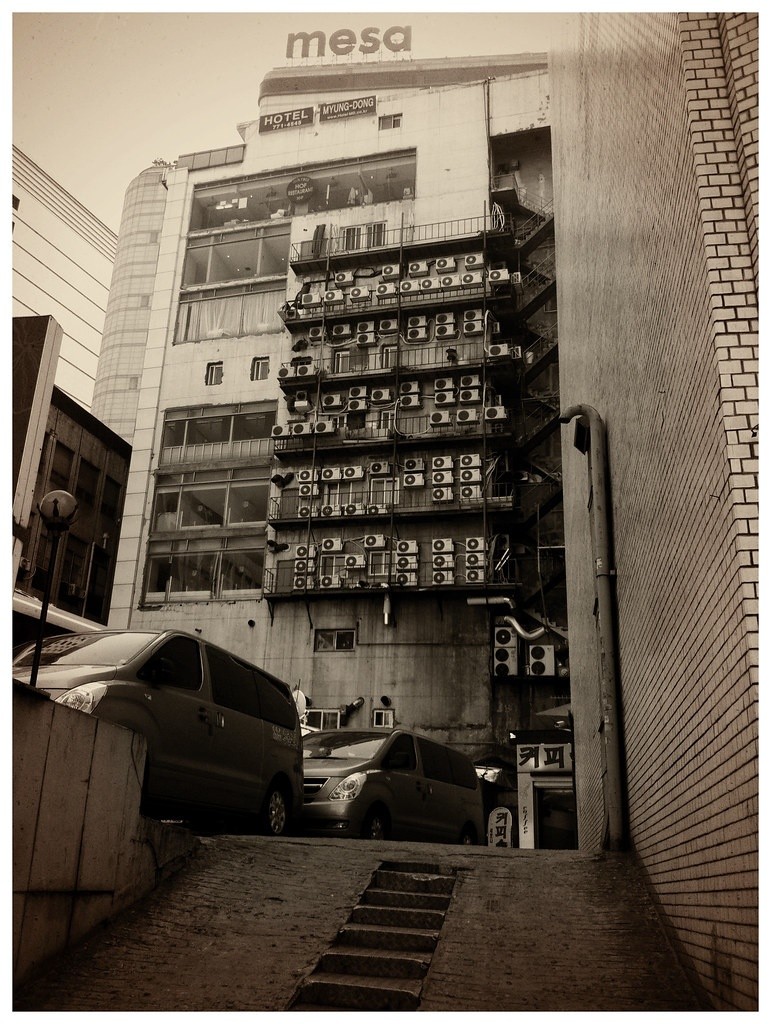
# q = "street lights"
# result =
<box><xmin>29</xmin><ymin>490</ymin><xmax>81</xmax><ymax>685</ymax></box>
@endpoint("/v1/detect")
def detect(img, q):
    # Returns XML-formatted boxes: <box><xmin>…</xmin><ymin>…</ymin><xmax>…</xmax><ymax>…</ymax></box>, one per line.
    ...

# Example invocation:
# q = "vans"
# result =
<box><xmin>302</xmin><ymin>726</ymin><xmax>486</xmax><ymax>846</ymax></box>
<box><xmin>13</xmin><ymin>627</ymin><xmax>305</xmax><ymax>838</ymax></box>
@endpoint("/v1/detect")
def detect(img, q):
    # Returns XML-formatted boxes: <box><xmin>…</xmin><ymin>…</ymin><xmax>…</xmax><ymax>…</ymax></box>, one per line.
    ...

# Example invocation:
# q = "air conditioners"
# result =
<box><xmin>271</xmin><ymin>252</ymin><xmax>522</xmax><ymax>591</ymax></box>
<box><xmin>492</xmin><ymin>626</ymin><xmax>554</xmax><ymax>677</ymax></box>
